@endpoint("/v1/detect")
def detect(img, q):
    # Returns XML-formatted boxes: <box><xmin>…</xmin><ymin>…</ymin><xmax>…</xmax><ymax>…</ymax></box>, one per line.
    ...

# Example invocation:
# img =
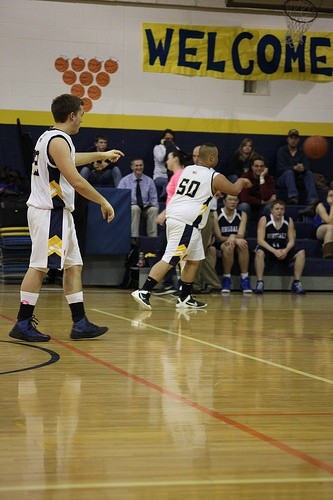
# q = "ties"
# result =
<box><xmin>135</xmin><ymin>178</ymin><xmax>144</xmax><ymax>212</ymax></box>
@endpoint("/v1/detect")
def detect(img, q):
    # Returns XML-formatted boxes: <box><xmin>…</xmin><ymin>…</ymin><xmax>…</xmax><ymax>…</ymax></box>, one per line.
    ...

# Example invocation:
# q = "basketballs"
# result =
<box><xmin>88</xmin><ymin>86</ymin><xmax>101</xmax><ymax>100</ymax></box>
<box><xmin>96</xmin><ymin>72</ymin><xmax>110</xmax><ymax>87</ymax></box>
<box><xmin>63</xmin><ymin>70</ymin><xmax>76</xmax><ymax>85</ymax></box>
<box><xmin>88</xmin><ymin>59</ymin><xmax>101</xmax><ymax>73</ymax></box>
<box><xmin>80</xmin><ymin>72</ymin><xmax>94</xmax><ymax>86</ymax></box>
<box><xmin>71</xmin><ymin>57</ymin><xmax>85</xmax><ymax>71</ymax></box>
<box><xmin>81</xmin><ymin>98</ymin><xmax>93</xmax><ymax>112</ymax></box>
<box><xmin>71</xmin><ymin>84</ymin><xmax>85</xmax><ymax>98</ymax></box>
<box><xmin>303</xmin><ymin>135</ymin><xmax>327</xmax><ymax>159</ymax></box>
<box><xmin>104</xmin><ymin>59</ymin><xmax>117</xmax><ymax>74</ymax></box>
<box><xmin>55</xmin><ymin>58</ymin><xmax>68</xmax><ymax>72</ymax></box>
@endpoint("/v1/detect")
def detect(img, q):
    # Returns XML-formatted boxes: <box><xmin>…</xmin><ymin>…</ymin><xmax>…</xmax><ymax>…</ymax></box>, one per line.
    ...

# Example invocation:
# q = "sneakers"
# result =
<box><xmin>256</xmin><ymin>281</ymin><xmax>264</xmax><ymax>294</ymax></box>
<box><xmin>175</xmin><ymin>295</ymin><xmax>207</xmax><ymax>308</ymax></box>
<box><xmin>132</xmin><ymin>311</ymin><xmax>151</xmax><ymax>329</ymax></box>
<box><xmin>175</xmin><ymin>309</ymin><xmax>207</xmax><ymax>322</ymax></box>
<box><xmin>220</xmin><ymin>275</ymin><xmax>230</xmax><ymax>293</ymax></box>
<box><xmin>131</xmin><ymin>288</ymin><xmax>152</xmax><ymax>312</ymax></box>
<box><xmin>9</xmin><ymin>314</ymin><xmax>50</xmax><ymax>341</ymax></box>
<box><xmin>153</xmin><ymin>284</ymin><xmax>177</xmax><ymax>296</ymax></box>
<box><xmin>291</xmin><ymin>281</ymin><xmax>305</xmax><ymax>295</ymax></box>
<box><xmin>239</xmin><ymin>277</ymin><xmax>253</xmax><ymax>292</ymax></box>
<box><xmin>69</xmin><ymin>318</ymin><xmax>108</xmax><ymax>339</ymax></box>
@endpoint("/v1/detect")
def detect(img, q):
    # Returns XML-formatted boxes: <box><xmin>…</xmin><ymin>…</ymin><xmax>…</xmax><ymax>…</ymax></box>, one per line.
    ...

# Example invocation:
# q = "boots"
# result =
<box><xmin>322</xmin><ymin>241</ymin><xmax>333</xmax><ymax>260</ymax></box>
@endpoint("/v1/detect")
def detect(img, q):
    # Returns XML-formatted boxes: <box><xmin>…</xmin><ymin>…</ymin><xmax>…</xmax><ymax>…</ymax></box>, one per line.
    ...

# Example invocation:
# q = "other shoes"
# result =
<box><xmin>288</xmin><ymin>198</ymin><xmax>297</xmax><ymax>204</ymax></box>
<box><xmin>309</xmin><ymin>200</ymin><xmax>317</xmax><ymax>206</ymax></box>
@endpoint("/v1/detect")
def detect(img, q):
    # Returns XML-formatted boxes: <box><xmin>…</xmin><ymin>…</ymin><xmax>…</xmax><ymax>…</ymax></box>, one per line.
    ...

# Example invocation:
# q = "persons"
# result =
<box><xmin>118</xmin><ymin>157</ymin><xmax>159</xmax><ymax>244</ymax></box>
<box><xmin>154</xmin><ymin>129</ymin><xmax>183</xmax><ymax>200</ymax></box>
<box><xmin>255</xmin><ymin>199</ymin><xmax>306</xmax><ymax>293</ymax></box>
<box><xmin>274</xmin><ymin>129</ymin><xmax>318</xmax><ymax>203</ymax></box>
<box><xmin>229</xmin><ymin>138</ymin><xmax>256</xmax><ymax>183</ymax></box>
<box><xmin>80</xmin><ymin>135</ymin><xmax>122</xmax><ymax>188</ymax></box>
<box><xmin>213</xmin><ymin>192</ymin><xmax>252</xmax><ymax>293</ymax></box>
<box><xmin>240</xmin><ymin>154</ymin><xmax>277</xmax><ymax>238</ymax></box>
<box><xmin>191</xmin><ymin>211</ymin><xmax>216</xmax><ymax>293</ymax></box>
<box><xmin>130</xmin><ymin>142</ymin><xmax>253</xmax><ymax>309</ymax></box>
<box><xmin>312</xmin><ymin>188</ymin><xmax>333</xmax><ymax>258</ymax></box>
<box><xmin>193</xmin><ymin>145</ymin><xmax>217</xmax><ymax>211</ymax></box>
<box><xmin>8</xmin><ymin>94</ymin><xmax>125</xmax><ymax>343</ymax></box>
<box><xmin>151</xmin><ymin>150</ymin><xmax>187</xmax><ymax>298</ymax></box>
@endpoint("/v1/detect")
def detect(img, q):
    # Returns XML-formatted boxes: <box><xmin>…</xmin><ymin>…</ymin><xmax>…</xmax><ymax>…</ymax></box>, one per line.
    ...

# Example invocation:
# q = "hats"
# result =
<box><xmin>288</xmin><ymin>129</ymin><xmax>298</xmax><ymax>138</ymax></box>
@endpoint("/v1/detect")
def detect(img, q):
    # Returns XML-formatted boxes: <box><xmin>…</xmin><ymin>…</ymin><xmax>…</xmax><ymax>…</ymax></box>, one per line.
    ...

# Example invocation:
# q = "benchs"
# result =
<box><xmin>129</xmin><ymin>202</ymin><xmax>333</xmax><ymax>289</ymax></box>
